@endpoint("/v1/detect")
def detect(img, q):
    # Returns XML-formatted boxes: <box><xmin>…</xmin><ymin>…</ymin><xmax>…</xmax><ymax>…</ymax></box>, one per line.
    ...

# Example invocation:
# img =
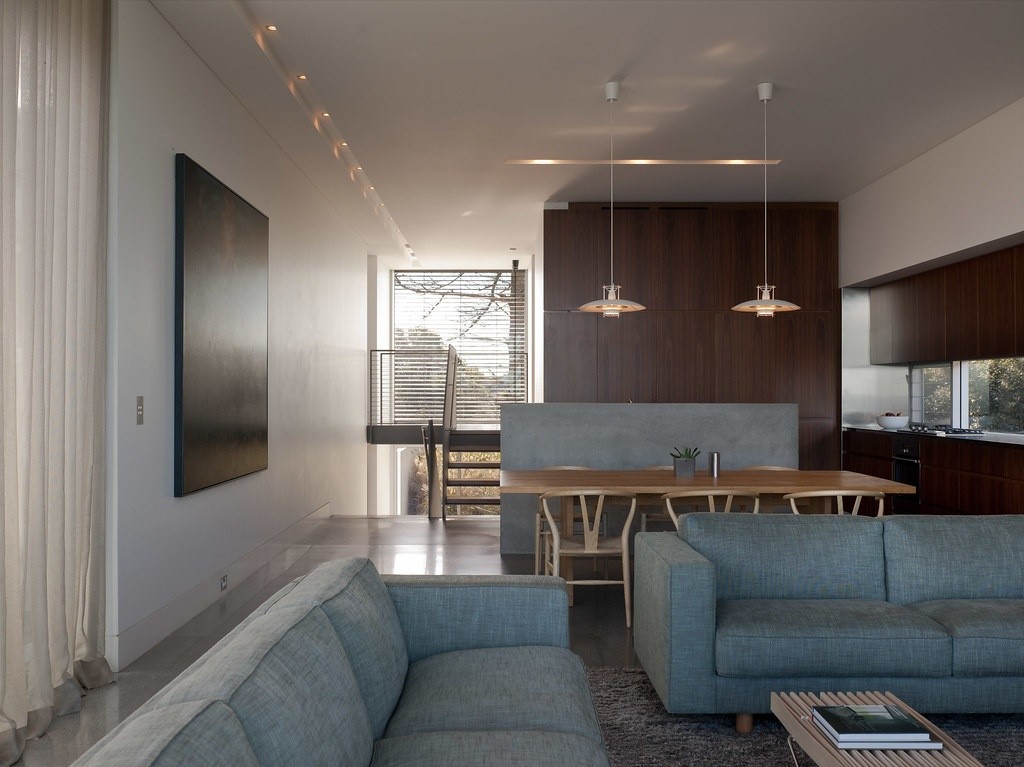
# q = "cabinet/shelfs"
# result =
<box><xmin>870</xmin><ymin>243</ymin><xmax>1024</xmax><ymax>366</ymax></box>
<box><xmin>843</xmin><ymin>430</ymin><xmax>1024</xmax><ymax>518</ymax></box>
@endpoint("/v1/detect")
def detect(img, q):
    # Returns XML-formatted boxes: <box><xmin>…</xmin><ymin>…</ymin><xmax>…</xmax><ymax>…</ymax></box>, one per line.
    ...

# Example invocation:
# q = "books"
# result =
<box><xmin>811</xmin><ymin>705</ymin><xmax>943</xmax><ymax>750</ymax></box>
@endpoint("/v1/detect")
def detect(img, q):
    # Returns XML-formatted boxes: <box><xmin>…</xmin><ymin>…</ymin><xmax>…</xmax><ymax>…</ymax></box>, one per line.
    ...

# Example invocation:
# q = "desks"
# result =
<box><xmin>499</xmin><ymin>469</ymin><xmax>915</xmax><ymax>607</ymax></box>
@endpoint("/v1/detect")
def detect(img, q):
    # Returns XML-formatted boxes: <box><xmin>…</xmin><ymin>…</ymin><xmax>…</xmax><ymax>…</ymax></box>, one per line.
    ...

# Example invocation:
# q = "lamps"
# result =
<box><xmin>731</xmin><ymin>83</ymin><xmax>801</xmax><ymax>318</ymax></box>
<box><xmin>578</xmin><ymin>81</ymin><xmax>646</xmax><ymax>319</ymax></box>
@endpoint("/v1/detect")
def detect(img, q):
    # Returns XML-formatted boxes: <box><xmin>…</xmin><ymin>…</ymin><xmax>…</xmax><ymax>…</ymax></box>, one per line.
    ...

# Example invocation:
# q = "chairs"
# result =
<box><xmin>661</xmin><ymin>488</ymin><xmax>760</xmax><ymax>535</ymax></box>
<box><xmin>784</xmin><ymin>490</ymin><xmax>886</xmax><ymax>516</ymax></box>
<box><xmin>537</xmin><ymin>490</ymin><xmax>637</xmax><ymax>628</ymax></box>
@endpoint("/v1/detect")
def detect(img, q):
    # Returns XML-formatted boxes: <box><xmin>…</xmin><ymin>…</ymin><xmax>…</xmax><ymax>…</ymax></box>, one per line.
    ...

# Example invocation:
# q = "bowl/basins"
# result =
<box><xmin>876</xmin><ymin>416</ymin><xmax>909</xmax><ymax>429</ymax></box>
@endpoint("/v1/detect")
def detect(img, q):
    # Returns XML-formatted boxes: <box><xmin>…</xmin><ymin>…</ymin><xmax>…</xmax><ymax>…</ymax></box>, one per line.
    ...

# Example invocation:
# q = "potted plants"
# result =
<box><xmin>670</xmin><ymin>443</ymin><xmax>700</xmax><ymax>476</ymax></box>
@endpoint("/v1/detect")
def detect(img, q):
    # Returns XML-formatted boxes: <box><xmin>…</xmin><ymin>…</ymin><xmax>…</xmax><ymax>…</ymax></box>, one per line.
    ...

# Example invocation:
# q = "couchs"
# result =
<box><xmin>633</xmin><ymin>512</ymin><xmax>1024</xmax><ymax>732</ymax></box>
<box><xmin>70</xmin><ymin>558</ymin><xmax>611</xmax><ymax>767</ymax></box>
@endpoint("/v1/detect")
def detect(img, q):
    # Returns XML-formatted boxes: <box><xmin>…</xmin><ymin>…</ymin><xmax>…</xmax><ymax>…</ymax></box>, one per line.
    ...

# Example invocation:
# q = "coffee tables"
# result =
<box><xmin>770</xmin><ymin>691</ymin><xmax>985</xmax><ymax>767</ymax></box>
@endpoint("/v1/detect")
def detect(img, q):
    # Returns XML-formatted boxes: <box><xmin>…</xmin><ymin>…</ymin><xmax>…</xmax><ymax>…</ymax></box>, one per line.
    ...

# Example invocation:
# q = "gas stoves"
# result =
<box><xmin>897</xmin><ymin>424</ymin><xmax>985</xmax><ymax>437</ymax></box>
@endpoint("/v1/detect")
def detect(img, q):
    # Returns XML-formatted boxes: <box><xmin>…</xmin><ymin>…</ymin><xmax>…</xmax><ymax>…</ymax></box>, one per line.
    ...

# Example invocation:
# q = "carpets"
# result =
<box><xmin>584</xmin><ymin>665</ymin><xmax>1024</xmax><ymax>767</ymax></box>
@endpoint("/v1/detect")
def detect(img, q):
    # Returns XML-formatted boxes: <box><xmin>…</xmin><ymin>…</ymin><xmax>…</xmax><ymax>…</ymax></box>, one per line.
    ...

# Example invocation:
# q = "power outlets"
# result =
<box><xmin>220</xmin><ymin>575</ymin><xmax>229</xmax><ymax>591</ymax></box>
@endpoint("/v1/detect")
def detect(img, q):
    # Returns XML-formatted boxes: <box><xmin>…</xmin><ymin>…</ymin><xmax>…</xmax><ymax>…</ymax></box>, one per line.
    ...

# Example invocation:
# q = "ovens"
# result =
<box><xmin>891</xmin><ymin>436</ymin><xmax>921</xmax><ymax>515</ymax></box>
